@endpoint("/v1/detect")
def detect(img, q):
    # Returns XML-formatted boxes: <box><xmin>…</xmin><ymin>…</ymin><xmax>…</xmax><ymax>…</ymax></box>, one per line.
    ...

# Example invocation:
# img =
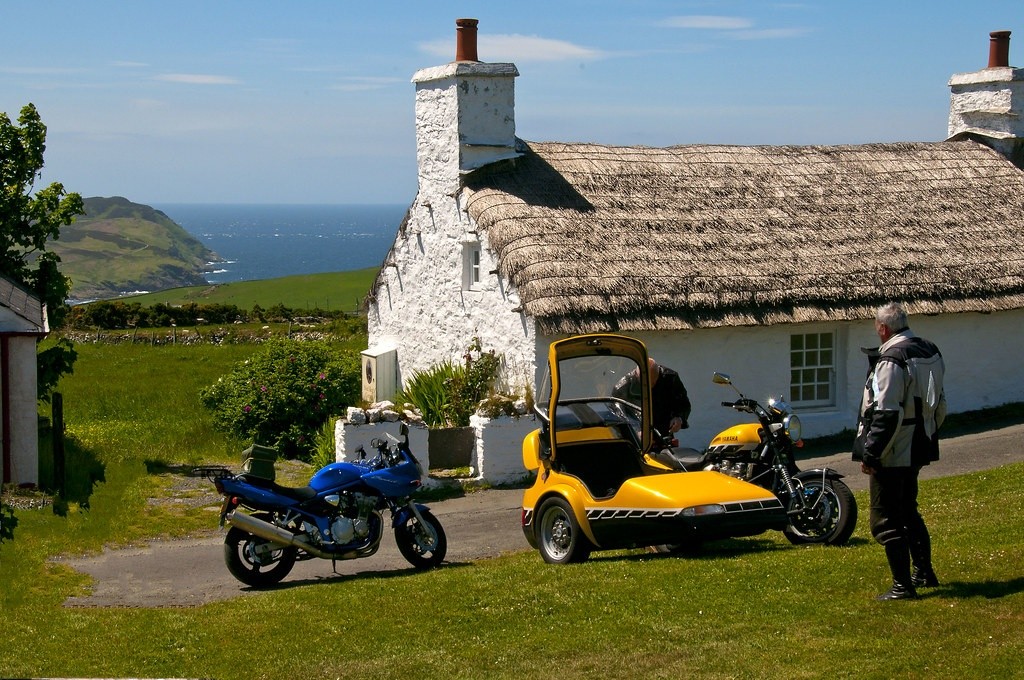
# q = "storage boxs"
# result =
<box><xmin>241</xmin><ymin>443</ymin><xmax>277</xmax><ymax>481</ymax></box>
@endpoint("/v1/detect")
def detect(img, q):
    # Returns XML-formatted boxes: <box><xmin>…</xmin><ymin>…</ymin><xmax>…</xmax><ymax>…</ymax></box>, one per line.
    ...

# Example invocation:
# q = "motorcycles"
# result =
<box><xmin>521</xmin><ymin>332</ymin><xmax>858</xmax><ymax>566</ymax></box>
<box><xmin>190</xmin><ymin>420</ymin><xmax>447</xmax><ymax>589</ymax></box>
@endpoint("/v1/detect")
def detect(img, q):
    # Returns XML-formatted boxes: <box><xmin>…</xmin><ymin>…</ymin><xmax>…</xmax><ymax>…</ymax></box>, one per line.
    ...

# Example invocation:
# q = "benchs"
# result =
<box><xmin>553</xmin><ymin>438</ymin><xmax>643</xmax><ymax>494</ymax></box>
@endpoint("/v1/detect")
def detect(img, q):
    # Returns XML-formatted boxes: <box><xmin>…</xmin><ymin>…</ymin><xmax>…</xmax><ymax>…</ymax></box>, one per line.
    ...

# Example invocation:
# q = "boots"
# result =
<box><xmin>903</xmin><ymin>514</ymin><xmax>940</xmax><ymax>587</ymax></box>
<box><xmin>878</xmin><ymin>543</ymin><xmax>917</xmax><ymax>600</ymax></box>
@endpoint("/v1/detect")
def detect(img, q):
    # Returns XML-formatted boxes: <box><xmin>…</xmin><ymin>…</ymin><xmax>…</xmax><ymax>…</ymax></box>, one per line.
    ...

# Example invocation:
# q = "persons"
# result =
<box><xmin>612</xmin><ymin>358</ymin><xmax>692</xmax><ymax>451</ymax></box>
<box><xmin>852</xmin><ymin>302</ymin><xmax>946</xmax><ymax>601</ymax></box>
